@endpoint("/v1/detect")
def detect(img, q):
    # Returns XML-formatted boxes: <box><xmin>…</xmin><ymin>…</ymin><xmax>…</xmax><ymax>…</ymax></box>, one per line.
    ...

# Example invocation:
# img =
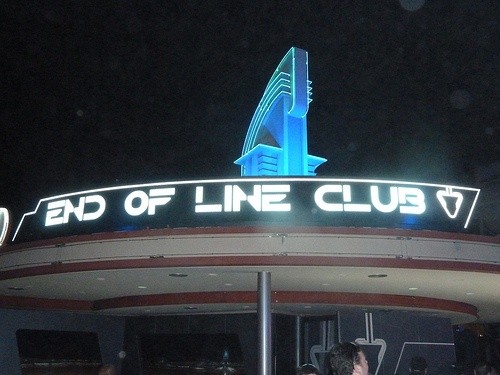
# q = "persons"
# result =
<box><xmin>97</xmin><ymin>365</ymin><xmax>116</xmax><ymax>374</ymax></box>
<box><xmin>326</xmin><ymin>343</ymin><xmax>370</xmax><ymax>375</ymax></box>
<box><xmin>474</xmin><ymin>363</ymin><xmax>496</xmax><ymax>374</ymax></box>
<box><xmin>298</xmin><ymin>365</ymin><xmax>319</xmax><ymax>373</ymax></box>
<box><xmin>407</xmin><ymin>357</ymin><xmax>427</xmax><ymax>375</ymax></box>
